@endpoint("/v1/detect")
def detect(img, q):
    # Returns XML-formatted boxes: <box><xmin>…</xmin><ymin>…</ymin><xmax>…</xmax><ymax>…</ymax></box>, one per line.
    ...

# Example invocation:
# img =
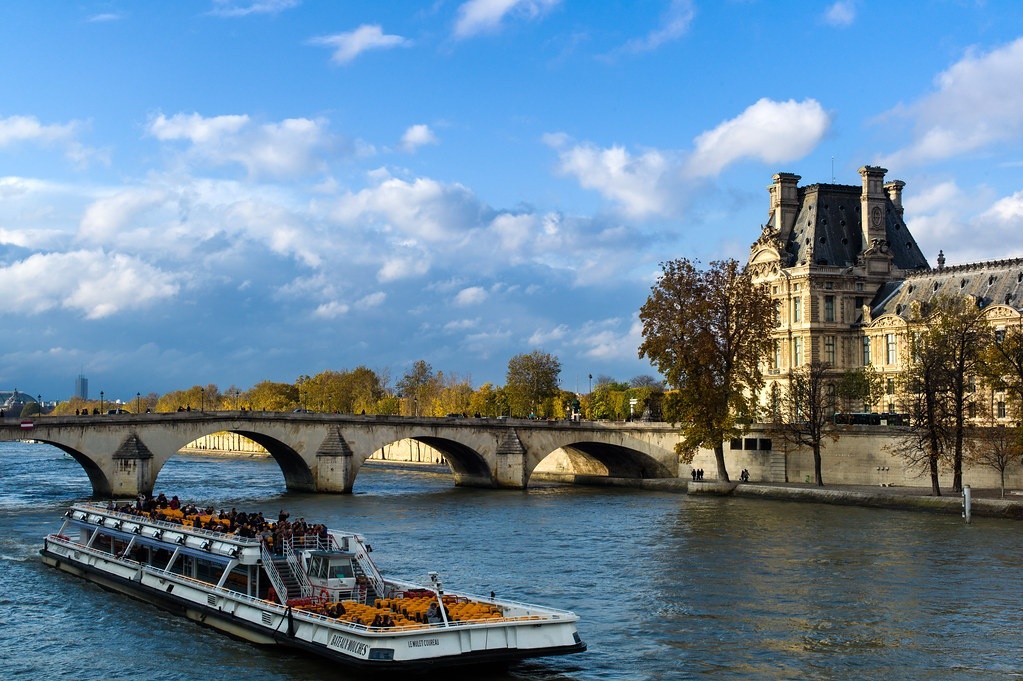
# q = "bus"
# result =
<box><xmin>833</xmin><ymin>412</ymin><xmax>910</xmax><ymax>427</ymax></box>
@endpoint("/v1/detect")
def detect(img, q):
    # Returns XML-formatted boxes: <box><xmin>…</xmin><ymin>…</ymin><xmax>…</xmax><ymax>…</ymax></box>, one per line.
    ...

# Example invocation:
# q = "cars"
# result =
<box><xmin>293</xmin><ymin>408</ymin><xmax>314</xmax><ymax>414</ymax></box>
<box><xmin>108</xmin><ymin>409</ymin><xmax>131</xmax><ymax>414</ymax></box>
<box><xmin>497</xmin><ymin>415</ymin><xmax>510</xmax><ymax>420</ymax></box>
<box><xmin>445</xmin><ymin>413</ymin><xmax>463</xmax><ymax>418</ymax></box>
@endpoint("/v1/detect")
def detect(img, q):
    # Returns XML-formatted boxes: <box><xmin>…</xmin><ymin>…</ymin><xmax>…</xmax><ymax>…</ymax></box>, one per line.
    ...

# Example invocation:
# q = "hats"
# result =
<box><xmin>259</xmin><ymin>512</ymin><xmax>263</xmax><ymax>515</ymax></box>
<box><xmin>159</xmin><ymin>493</ymin><xmax>165</xmax><ymax>496</ymax></box>
<box><xmin>137</xmin><ymin>491</ymin><xmax>141</xmax><ymax>494</ymax></box>
<box><xmin>173</xmin><ymin>496</ymin><xmax>178</xmax><ymax>501</ymax></box>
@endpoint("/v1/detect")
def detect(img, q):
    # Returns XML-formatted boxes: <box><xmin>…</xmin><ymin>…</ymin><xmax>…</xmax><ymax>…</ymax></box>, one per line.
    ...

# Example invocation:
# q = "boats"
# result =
<box><xmin>38</xmin><ymin>494</ymin><xmax>588</xmax><ymax>673</ymax></box>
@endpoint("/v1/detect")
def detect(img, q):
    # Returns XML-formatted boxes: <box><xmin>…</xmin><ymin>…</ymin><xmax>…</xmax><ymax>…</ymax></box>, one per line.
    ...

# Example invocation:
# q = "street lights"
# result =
<box><xmin>201</xmin><ymin>387</ymin><xmax>204</xmax><ymax>412</ymax></box>
<box><xmin>100</xmin><ymin>391</ymin><xmax>104</xmax><ymax>414</ymax></box>
<box><xmin>303</xmin><ymin>391</ymin><xmax>307</xmax><ymax>413</ymax></box>
<box><xmin>137</xmin><ymin>392</ymin><xmax>140</xmax><ymax>413</ymax></box>
<box><xmin>329</xmin><ymin>395</ymin><xmax>331</xmax><ymax>413</ymax></box>
<box><xmin>37</xmin><ymin>394</ymin><xmax>41</xmax><ymax>417</ymax></box>
<box><xmin>235</xmin><ymin>390</ymin><xmax>239</xmax><ymax>410</ymax></box>
<box><xmin>414</xmin><ymin>398</ymin><xmax>417</xmax><ymax>416</ymax></box>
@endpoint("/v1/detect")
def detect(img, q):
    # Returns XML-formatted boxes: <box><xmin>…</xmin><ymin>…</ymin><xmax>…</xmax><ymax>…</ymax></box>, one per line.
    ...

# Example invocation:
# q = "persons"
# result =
<box><xmin>462</xmin><ymin>411</ymin><xmax>481</xmax><ymax>418</ymax></box>
<box><xmin>87</xmin><ymin>492</ymin><xmax>327</xmax><ymax>556</ymax></box>
<box><xmin>329</xmin><ymin>601</ymin><xmax>452</xmax><ymax>632</ymax></box>
<box><xmin>691</xmin><ymin>468</ymin><xmax>704</xmax><ymax>480</ymax></box>
<box><xmin>741</xmin><ymin>469</ymin><xmax>750</xmax><ymax>482</ymax></box>
<box><xmin>75</xmin><ymin>404</ymin><xmax>366</xmax><ymax>415</ymax></box>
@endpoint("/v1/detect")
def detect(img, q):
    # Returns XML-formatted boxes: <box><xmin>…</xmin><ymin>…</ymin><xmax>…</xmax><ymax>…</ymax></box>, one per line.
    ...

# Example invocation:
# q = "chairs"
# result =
<box><xmin>263</xmin><ymin>590</ymin><xmax>548</xmax><ymax>633</ymax></box>
<box><xmin>134</xmin><ymin>506</ymin><xmax>305</xmax><ymax>550</ymax></box>
